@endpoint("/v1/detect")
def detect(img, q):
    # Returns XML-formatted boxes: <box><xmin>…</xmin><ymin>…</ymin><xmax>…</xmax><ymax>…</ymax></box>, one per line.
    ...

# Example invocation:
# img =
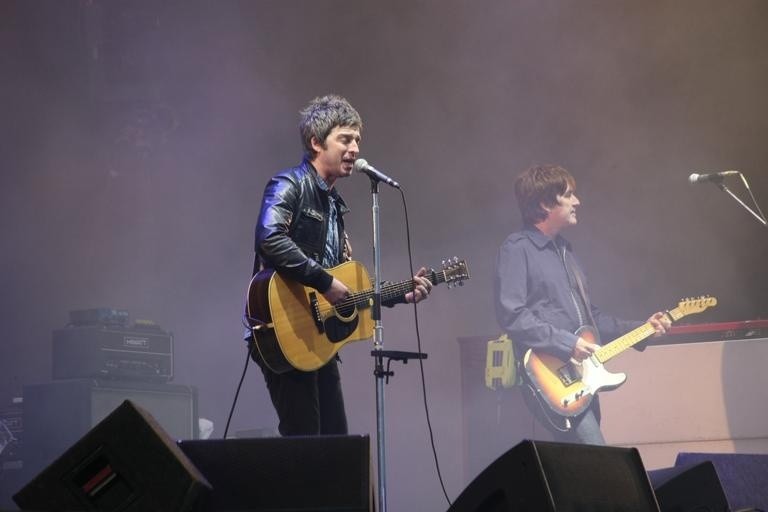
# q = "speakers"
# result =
<box><xmin>20</xmin><ymin>383</ymin><xmax>199</xmax><ymax>483</ymax></box>
<box><xmin>445</xmin><ymin>438</ymin><xmax>661</xmax><ymax>512</ymax></box>
<box><xmin>653</xmin><ymin>458</ymin><xmax>768</xmax><ymax>512</ymax></box>
<box><xmin>177</xmin><ymin>433</ymin><xmax>377</xmax><ymax>512</ymax></box>
<box><xmin>12</xmin><ymin>400</ymin><xmax>213</xmax><ymax>512</ymax></box>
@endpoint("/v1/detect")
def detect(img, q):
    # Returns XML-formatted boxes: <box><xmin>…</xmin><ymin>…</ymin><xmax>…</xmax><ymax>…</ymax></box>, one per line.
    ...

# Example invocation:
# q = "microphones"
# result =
<box><xmin>688</xmin><ymin>171</ymin><xmax>740</xmax><ymax>185</ymax></box>
<box><xmin>354</xmin><ymin>158</ymin><xmax>401</xmax><ymax>189</ymax></box>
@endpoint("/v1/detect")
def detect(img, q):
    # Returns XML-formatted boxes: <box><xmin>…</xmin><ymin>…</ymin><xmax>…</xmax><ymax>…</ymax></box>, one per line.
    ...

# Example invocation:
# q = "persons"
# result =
<box><xmin>491</xmin><ymin>161</ymin><xmax>673</xmax><ymax>447</ymax></box>
<box><xmin>241</xmin><ymin>92</ymin><xmax>433</xmax><ymax>438</ymax></box>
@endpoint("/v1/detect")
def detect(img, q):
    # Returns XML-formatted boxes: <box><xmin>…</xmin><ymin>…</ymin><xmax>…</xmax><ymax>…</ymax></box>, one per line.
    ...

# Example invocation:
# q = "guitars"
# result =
<box><xmin>521</xmin><ymin>295</ymin><xmax>717</xmax><ymax>418</ymax></box>
<box><xmin>247</xmin><ymin>256</ymin><xmax>471</xmax><ymax>375</ymax></box>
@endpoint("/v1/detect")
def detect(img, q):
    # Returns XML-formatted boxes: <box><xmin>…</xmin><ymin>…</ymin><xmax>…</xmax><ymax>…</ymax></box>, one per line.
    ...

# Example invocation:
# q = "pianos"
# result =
<box><xmin>655</xmin><ymin>320</ymin><xmax>767</xmax><ymax>341</ymax></box>
